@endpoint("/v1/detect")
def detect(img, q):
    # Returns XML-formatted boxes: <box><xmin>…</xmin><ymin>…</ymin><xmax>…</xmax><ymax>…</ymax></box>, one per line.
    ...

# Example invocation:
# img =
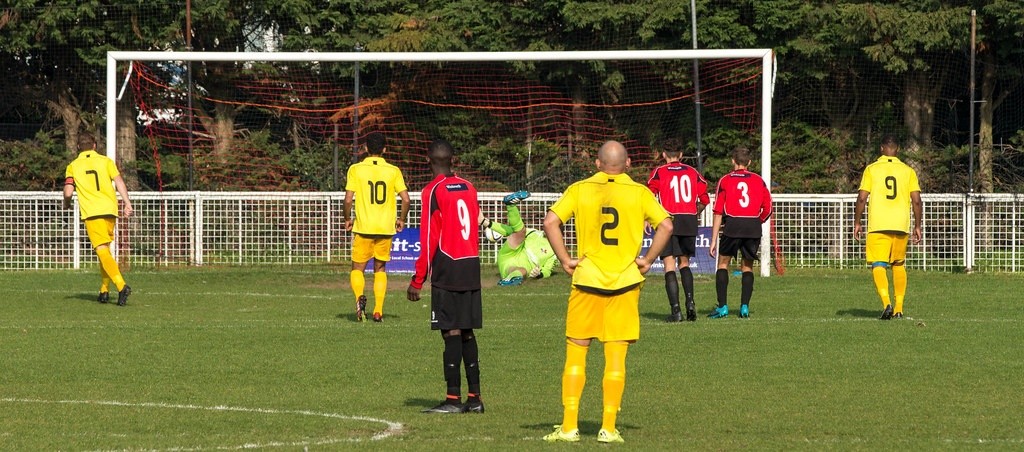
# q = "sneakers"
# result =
<box><xmin>892</xmin><ymin>312</ymin><xmax>903</xmax><ymax>320</ymax></box>
<box><xmin>503</xmin><ymin>191</ymin><xmax>530</xmax><ymax>205</ymax></box>
<box><xmin>740</xmin><ymin>305</ymin><xmax>750</xmax><ymax>319</ymax></box>
<box><xmin>117</xmin><ymin>285</ymin><xmax>132</xmax><ymax>306</ymax></box>
<box><xmin>462</xmin><ymin>401</ymin><xmax>484</xmax><ymax>414</ymax></box>
<box><xmin>542</xmin><ymin>424</ymin><xmax>580</xmax><ymax>442</ymax></box>
<box><xmin>98</xmin><ymin>291</ymin><xmax>110</xmax><ymax>303</ymax></box>
<box><xmin>597</xmin><ymin>429</ymin><xmax>624</xmax><ymax>443</ymax></box>
<box><xmin>706</xmin><ymin>305</ymin><xmax>729</xmax><ymax>318</ymax></box>
<box><xmin>686</xmin><ymin>302</ymin><xmax>697</xmax><ymax>321</ymax></box>
<box><xmin>356</xmin><ymin>295</ymin><xmax>368</xmax><ymax>322</ymax></box>
<box><xmin>665</xmin><ymin>313</ymin><xmax>683</xmax><ymax>323</ymax></box>
<box><xmin>421</xmin><ymin>401</ymin><xmax>463</xmax><ymax>413</ymax></box>
<box><xmin>497</xmin><ymin>276</ymin><xmax>523</xmax><ymax>287</ymax></box>
<box><xmin>373</xmin><ymin>312</ymin><xmax>384</xmax><ymax>323</ymax></box>
<box><xmin>878</xmin><ymin>305</ymin><xmax>894</xmax><ymax>319</ymax></box>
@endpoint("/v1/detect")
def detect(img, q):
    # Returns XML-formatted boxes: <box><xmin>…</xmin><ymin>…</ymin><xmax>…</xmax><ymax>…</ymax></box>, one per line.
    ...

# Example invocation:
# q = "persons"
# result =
<box><xmin>644</xmin><ymin>139</ymin><xmax>710</xmax><ymax>323</ymax></box>
<box><xmin>543</xmin><ymin>140</ymin><xmax>673</xmax><ymax>442</ymax></box>
<box><xmin>343</xmin><ymin>133</ymin><xmax>410</xmax><ymax>322</ymax></box>
<box><xmin>854</xmin><ymin>134</ymin><xmax>922</xmax><ymax>320</ymax></box>
<box><xmin>406</xmin><ymin>141</ymin><xmax>484</xmax><ymax>415</ymax></box>
<box><xmin>64</xmin><ymin>131</ymin><xmax>133</xmax><ymax>306</ymax></box>
<box><xmin>706</xmin><ymin>147</ymin><xmax>773</xmax><ymax>319</ymax></box>
<box><xmin>478</xmin><ymin>190</ymin><xmax>564</xmax><ymax>285</ymax></box>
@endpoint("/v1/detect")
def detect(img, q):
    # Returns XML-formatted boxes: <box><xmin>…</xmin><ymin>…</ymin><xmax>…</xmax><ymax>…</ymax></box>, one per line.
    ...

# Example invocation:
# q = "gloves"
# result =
<box><xmin>529</xmin><ymin>267</ymin><xmax>542</xmax><ymax>278</ymax></box>
<box><xmin>478</xmin><ymin>209</ymin><xmax>490</xmax><ymax>227</ymax></box>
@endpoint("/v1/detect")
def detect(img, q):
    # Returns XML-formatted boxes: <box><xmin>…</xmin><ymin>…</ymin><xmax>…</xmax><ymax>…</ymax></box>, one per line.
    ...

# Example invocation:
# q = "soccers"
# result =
<box><xmin>485</xmin><ymin>227</ymin><xmax>503</xmax><ymax>242</ymax></box>
